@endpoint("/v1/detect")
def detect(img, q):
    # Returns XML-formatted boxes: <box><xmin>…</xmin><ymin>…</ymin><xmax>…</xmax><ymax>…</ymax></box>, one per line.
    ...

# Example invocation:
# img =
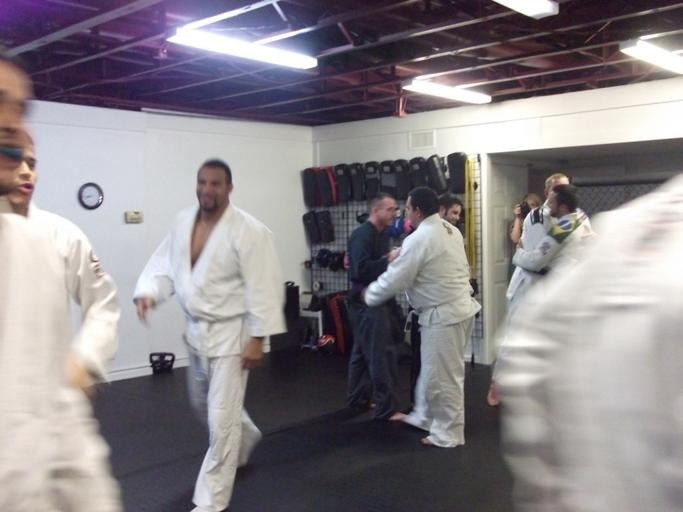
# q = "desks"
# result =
<box><xmin>299</xmin><ymin>309</ymin><xmax>323</xmax><ymax>351</ymax></box>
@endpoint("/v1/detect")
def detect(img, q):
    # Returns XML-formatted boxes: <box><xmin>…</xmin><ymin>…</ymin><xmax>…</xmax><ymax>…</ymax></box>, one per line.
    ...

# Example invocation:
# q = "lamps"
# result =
<box><xmin>164</xmin><ymin>27</ymin><xmax>318</xmax><ymax>70</ymax></box>
<box><xmin>618</xmin><ymin>39</ymin><xmax>683</xmax><ymax>75</ymax></box>
<box><xmin>402</xmin><ymin>79</ymin><xmax>493</xmax><ymax>104</ymax></box>
<box><xmin>490</xmin><ymin>0</ymin><xmax>560</xmax><ymax>20</ymax></box>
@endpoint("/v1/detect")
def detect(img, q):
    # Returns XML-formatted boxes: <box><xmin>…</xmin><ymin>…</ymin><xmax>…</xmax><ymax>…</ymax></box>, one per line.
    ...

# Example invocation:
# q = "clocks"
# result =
<box><xmin>78</xmin><ymin>184</ymin><xmax>103</xmax><ymax>209</ymax></box>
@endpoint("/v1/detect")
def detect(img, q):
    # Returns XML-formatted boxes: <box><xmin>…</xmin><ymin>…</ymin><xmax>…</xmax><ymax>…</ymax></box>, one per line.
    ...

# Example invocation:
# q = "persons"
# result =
<box><xmin>0</xmin><ymin>55</ymin><xmax>122</xmax><ymax>511</ymax></box>
<box><xmin>485</xmin><ymin>173</ymin><xmax>571</xmax><ymax>407</ymax></box>
<box><xmin>507</xmin><ymin>193</ymin><xmax>542</xmax><ymax>245</ymax></box>
<box><xmin>359</xmin><ymin>184</ymin><xmax>484</xmax><ymax>449</ymax></box>
<box><xmin>129</xmin><ymin>156</ymin><xmax>288</xmax><ymax>511</ymax></box>
<box><xmin>399</xmin><ymin>191</ymin><xmax>465</xmax><ymax>411</ymax></box>
<box><xmin>511</xmin><ymin>182</ymin><xmax>596</xmax><ymax>273</ymax></box>
<box><xmin>344</xmin><ymin>193</ymin><xmax>408</xmax><ymax>421</ymax></box>
<box><xmin>0</xmin><ymin>127</ymin><xmax>122</xmax><ymax>403</ymax></box>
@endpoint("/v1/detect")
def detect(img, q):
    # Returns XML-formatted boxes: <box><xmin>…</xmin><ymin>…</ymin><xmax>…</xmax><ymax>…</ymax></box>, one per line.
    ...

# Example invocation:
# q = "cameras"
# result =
<box><xmin>519</xmin><ymin>202</ymin><xmax>530</xmax><ymax>218</ymax></box>
